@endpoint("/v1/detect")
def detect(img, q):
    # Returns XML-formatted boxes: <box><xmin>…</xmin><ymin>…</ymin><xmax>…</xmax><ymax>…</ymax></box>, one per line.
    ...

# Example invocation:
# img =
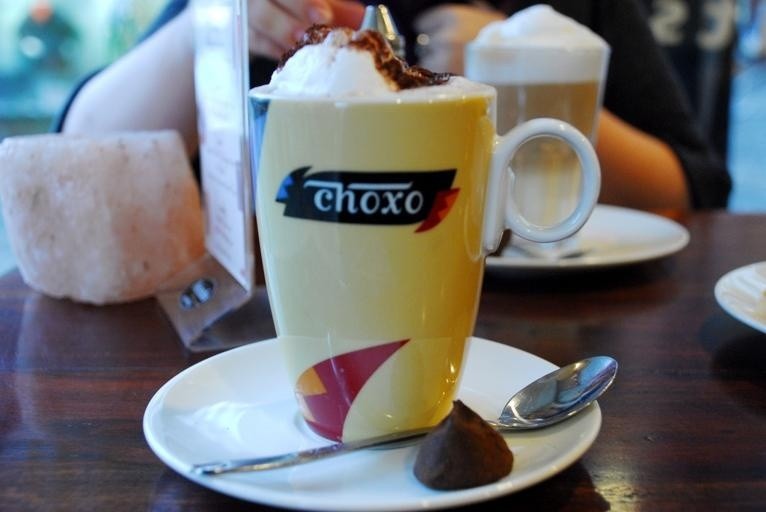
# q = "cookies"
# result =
<box><xmin>413</xmin><ymin>398</ymin><xmax>513</xmax><ymax>492</ymax></box>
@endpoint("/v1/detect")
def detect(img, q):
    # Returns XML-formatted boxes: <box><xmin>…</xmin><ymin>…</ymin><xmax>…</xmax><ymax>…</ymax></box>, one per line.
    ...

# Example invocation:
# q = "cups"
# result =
<box><xmin>462</xmin><ymin>44</ymin><xmax>611</xmax><ymax>260</ymax></box>
<box><xmin>247</xmin><ymin>83</ymin><xmax>603</xmax><ymax>450</ymax></box>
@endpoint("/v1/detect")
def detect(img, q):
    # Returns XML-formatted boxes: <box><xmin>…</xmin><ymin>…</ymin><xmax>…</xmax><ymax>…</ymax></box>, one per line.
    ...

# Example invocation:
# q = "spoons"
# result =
<box><xmin>188</xmin><ymin>355</ymin><xmax>617</xmax><ymax>480</ymax></box>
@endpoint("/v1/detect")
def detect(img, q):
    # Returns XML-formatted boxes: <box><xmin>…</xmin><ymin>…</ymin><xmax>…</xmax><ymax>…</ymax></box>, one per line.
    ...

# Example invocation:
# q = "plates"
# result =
<box><xmin>486</xmin><ymin>204</ymin><xmax>695</xmax><ymax>277</ymax></box>
<box><xmin>715</xmin><ymin>262</ymin><xmax>766</xmax><ymax>333</ymax></box>
<box><xmin>147</xmin><ymin>334</ymin><xmax>603</xmax><ymax>511</ymax></box>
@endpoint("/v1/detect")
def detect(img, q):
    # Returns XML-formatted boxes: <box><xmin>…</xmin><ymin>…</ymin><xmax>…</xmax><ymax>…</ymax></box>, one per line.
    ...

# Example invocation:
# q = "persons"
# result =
<box><xmin>47</xmin><ymin>1</ymin><xmax>728</xmax><ymax>213</ymax></box>
